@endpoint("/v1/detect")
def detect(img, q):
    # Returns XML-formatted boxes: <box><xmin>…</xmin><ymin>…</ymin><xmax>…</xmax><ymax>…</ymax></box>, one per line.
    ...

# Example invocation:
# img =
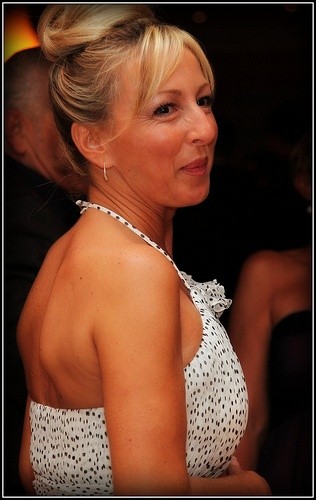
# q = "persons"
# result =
<box><xmin>2</xmin><ymin>45</ymin><xmax>82</xmax><ymax>497</ymax></box>
<box><xmin>17</xmin><ymin>5</ymin><xmax>273</xmax><ymax>498</ymax></box>
<box><xmin>233</xmin><ymin>176</ymin><xmax>316</xmax><ymax>499</ymax></box>
